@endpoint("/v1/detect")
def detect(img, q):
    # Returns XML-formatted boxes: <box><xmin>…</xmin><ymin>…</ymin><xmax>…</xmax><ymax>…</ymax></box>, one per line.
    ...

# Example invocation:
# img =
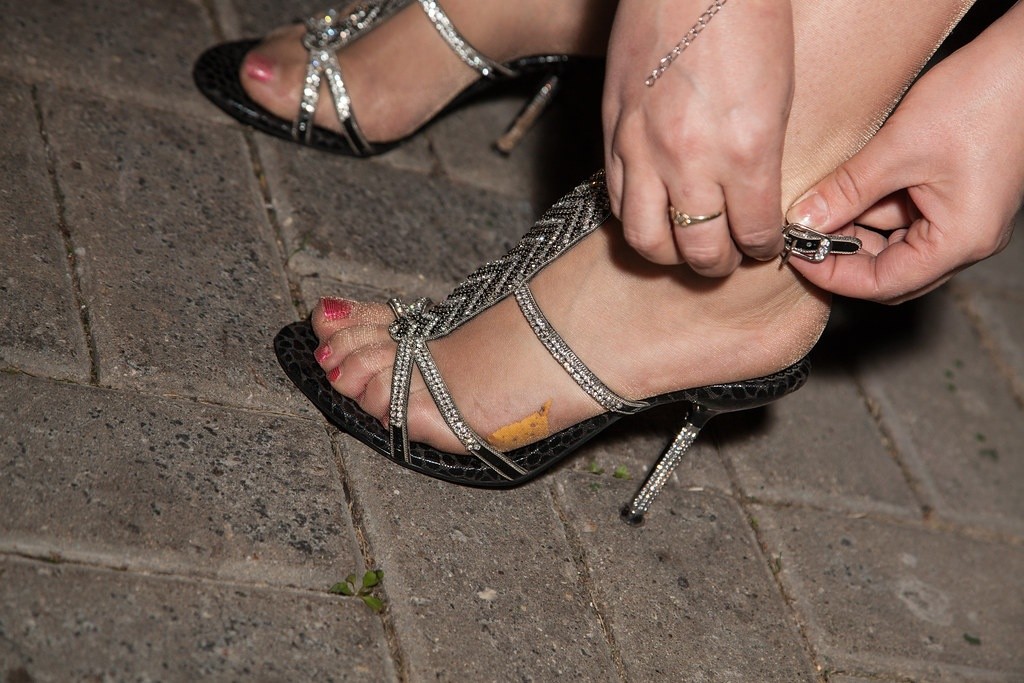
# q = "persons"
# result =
<box><xmin>193</xmin><ymin>0</ymin><xmax>1024</xmax><ymax>528</ymax></box>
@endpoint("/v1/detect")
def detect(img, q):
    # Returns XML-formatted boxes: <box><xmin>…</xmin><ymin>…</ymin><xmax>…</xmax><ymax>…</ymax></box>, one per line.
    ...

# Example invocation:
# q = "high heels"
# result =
<box><xmin>191</xmin><ymin>0</ymin><xmax>604</xmax><ymax>156</ymax></box>
<box><xmin>272</xmin><ymin>166</ymin><xmax>863</xmax><ymax>528</ymax></box>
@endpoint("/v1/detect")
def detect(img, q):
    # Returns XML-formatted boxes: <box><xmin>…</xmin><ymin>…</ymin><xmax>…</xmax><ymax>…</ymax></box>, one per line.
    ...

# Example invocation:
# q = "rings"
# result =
<box><xmin>669</xmin><ymin>208</ymin><xmax>726</xmax><ymax>226</ymax></box>
